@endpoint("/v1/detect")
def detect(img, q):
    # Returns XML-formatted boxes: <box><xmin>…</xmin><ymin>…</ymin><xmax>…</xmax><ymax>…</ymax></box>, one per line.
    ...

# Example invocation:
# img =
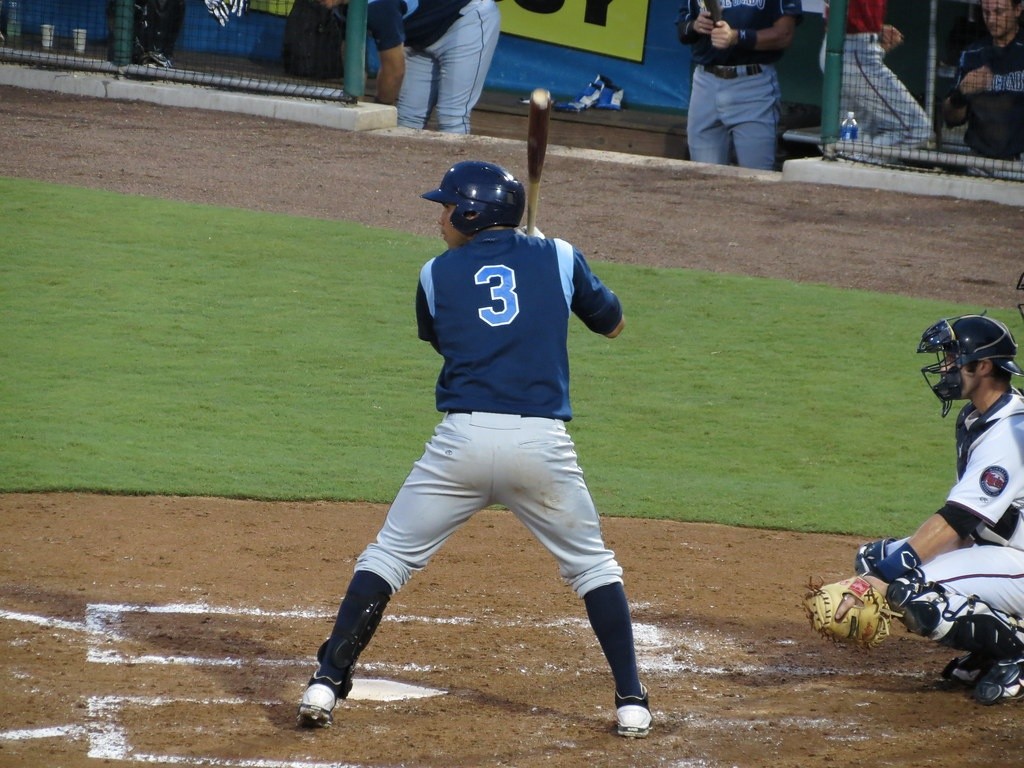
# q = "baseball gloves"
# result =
<box><xmin>795</xmin><ymin>573</ymin><xmax>906</xmax><ymax>659</ymax></box>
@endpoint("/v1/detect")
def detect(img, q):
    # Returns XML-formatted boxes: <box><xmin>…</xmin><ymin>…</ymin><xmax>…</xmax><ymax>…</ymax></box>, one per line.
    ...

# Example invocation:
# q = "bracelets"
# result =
<box><xmin>738</xmin><ymin>28</ymin><xmax>757</xmax><ymax>46</ymax></box>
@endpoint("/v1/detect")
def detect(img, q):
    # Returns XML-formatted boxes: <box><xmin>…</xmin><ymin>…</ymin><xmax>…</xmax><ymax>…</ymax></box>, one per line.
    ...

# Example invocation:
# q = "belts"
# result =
<box><xmin>704</xmin><ymin>64</ymin><xmax>761</xmax><ymax>79</ymax></box>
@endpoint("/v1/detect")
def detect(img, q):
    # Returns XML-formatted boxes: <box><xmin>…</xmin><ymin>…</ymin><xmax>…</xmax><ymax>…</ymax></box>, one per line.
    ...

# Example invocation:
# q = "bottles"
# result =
<box><xmin>840</xmin><ymin>111</ymin><xmax>858</xmax><ymax>158</ymax></box>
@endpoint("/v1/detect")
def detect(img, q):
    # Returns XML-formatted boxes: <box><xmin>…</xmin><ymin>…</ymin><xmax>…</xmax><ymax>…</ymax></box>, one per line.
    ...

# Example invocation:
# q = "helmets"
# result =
<box><xmin>917</xmin><ymin>311</ymin><xmax>1023</xmax><ymax>418</ymax></box>
<box><xmin>420</xmin><ymin>160</ymin><xmax>525</xmax><ymax>236</ymax></box>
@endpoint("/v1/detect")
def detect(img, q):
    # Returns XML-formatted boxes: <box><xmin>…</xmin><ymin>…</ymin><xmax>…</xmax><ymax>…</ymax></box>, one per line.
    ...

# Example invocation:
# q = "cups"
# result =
<box><xmin>40</xmin><ymin>24</ymin><xmax>55</xmax><ymax>47</ymax></box>
<box><xmin>72</xmin><ymin>29</ymin><xmax>87</xmax><ymax>54</ymax></box>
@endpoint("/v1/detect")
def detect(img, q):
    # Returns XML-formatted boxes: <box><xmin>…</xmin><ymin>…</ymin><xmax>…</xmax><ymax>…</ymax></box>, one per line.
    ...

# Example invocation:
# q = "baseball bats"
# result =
<box><xmin>524</xmin><ymin>87</ymin><xmax>553</xmax><ymax>237</ymax></box>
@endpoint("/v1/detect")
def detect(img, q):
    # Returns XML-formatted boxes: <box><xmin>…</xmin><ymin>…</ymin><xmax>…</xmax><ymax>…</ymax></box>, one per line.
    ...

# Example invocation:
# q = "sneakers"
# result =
<box><xmin>298</xmin><ymin>668</ymin><xmax>342</xmax><ymax>728</ymax></box>
<box><xmin>615</xmin><ymin>684</ymin><xmax>653</xmax><ymax>739</ymax></box>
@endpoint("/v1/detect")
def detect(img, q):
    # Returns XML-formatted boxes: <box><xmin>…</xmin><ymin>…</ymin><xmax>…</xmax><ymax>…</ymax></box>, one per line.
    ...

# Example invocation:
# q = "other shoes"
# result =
<box><xmin>934</xmin><ymin>652</ymin><xmax>996</xmax><ymax>692</ymax></box>
<box><xmin>140</xmin><ymin>51</ymin><xmax>171</xmax><ymax>70</ymax></box>
<box><xmin>973</xmin><ymin>657</ymin><xmax>1024</xmax><ymax>705</ymax></box>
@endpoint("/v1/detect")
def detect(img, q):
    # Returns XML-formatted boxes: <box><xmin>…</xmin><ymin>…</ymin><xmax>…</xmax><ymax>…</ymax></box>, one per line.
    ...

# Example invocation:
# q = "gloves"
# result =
<box><xmin>204</xmin><ymin>0</ymin><xmax>230</xmax><ymax>27</ymax></box>
<box><xmin>228</xmin><ymin>0</ymin><xmax>249</xmax><ymax>18</ymax></box>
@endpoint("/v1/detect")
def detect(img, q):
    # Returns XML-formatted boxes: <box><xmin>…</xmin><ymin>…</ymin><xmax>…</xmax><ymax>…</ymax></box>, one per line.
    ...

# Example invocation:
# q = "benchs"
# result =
<box><xmin>0</xmin><ymin>29</ymin><xmax>692</xmax><ymax>162</ymax></box>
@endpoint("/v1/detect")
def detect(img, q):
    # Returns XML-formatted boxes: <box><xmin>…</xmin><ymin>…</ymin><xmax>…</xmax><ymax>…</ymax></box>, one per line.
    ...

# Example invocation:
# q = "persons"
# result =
<box><xmin>290</xmin><ymin>159</ymin><xmax>652</xmax><ymax>741</ymax></box>
<box><xmin>835</xmin><ymin>320</ymin><xmax>1024</xmax><ymax>708</ymax></box>
<box><xmin>313</xmin><ymin>0</ymin><xmax>503</xmax><ymax>140</ymax></box>
<box><xmin>820</xmin><ymin>0</ymin><xmax>1024</xmax><ymax>182</ymax></box>
<box><xmin>675</xmin><ymin>0</ymin><xmax>803</xmax><ymax>174</ymax></box>
<box><xmin>104</xmin><ymin>0</ymin><xmax>186</xmax><ymax>67</ymax></box>
